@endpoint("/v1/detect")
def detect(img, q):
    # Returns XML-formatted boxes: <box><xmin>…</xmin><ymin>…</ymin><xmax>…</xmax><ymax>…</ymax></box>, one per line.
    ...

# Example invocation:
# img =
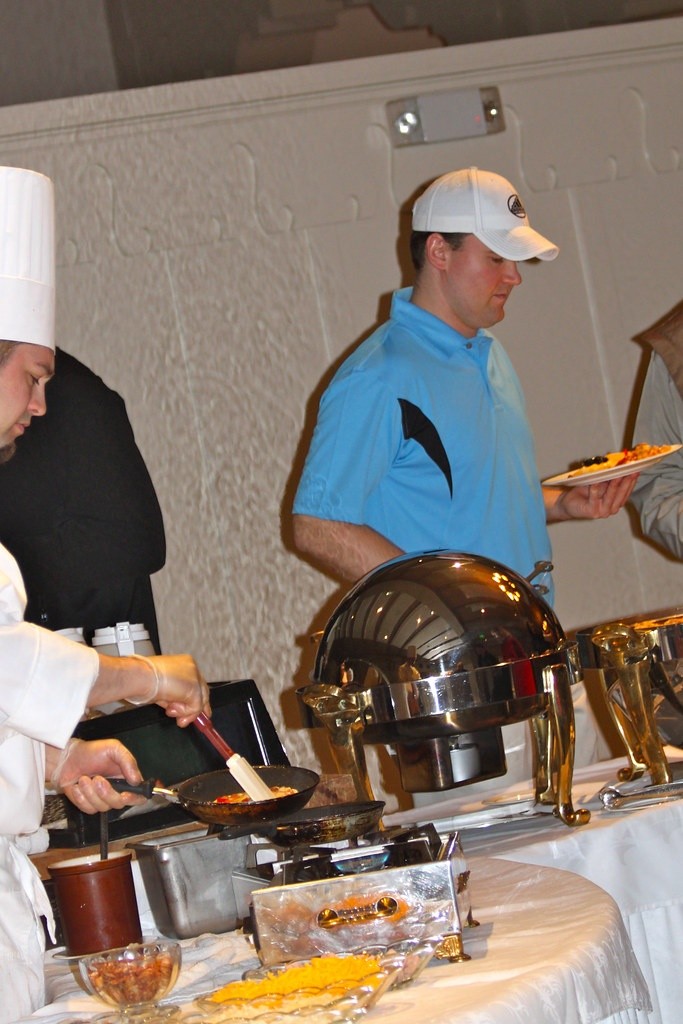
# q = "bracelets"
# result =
<box><xmin>122</xmin><ymin>650</ymin><xmax>159</xmax><ymax>707</ymax></box>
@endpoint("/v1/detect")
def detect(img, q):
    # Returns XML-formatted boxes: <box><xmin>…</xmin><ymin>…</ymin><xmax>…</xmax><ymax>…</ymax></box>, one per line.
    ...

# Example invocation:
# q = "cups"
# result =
<box><xmin>92</xmin><ymin>621</ymin><xmax>156</xmax><ymax>656</ymax></box>
<box><xmin>53</xmin><ymin>628</ymin><xmax>89</xmax><ymax>646</ymax></box>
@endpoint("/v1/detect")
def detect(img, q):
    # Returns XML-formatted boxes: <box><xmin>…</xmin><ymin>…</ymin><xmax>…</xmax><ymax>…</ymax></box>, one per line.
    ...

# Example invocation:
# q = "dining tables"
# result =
<box><xmin>0</xmin><ymin>669</ymin><xmax>682</xmax><ymax>1024</ymax></box>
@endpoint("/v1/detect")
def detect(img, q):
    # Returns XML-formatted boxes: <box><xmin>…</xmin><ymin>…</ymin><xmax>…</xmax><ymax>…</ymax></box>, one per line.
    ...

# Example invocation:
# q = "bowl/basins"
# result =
<box><xmin>53</xmin><ymin>946</ymin><xmax>147</xmax><ymax>996</ymax></box>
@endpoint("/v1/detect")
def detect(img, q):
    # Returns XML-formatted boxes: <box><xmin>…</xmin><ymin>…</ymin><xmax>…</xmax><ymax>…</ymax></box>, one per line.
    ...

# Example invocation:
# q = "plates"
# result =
<box><xmin>165</xmin><ymin>985</ymin><xmax>374</xmax><ymax>1024</ymax></box>
<box><xmin>192</xmin><ymin>936</ymin><xmax>444</xmax><ymax>1011</ymax></box>
<box><xmin>540</xmin><ymin>445</ymin><xmax>682</xmax><ymax>486</ymax></box>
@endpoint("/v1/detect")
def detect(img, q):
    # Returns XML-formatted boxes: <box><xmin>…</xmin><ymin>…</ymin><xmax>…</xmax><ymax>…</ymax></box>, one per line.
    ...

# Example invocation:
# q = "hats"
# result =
<box><xmin>0</xmin><ymin>167</ymin><xmax>58</xmax><ymax>355</ymax></box>
<box><xmin>412</xmin><ymin>165</ymin><xmax>561</xmax><ymax>267</ymax></box>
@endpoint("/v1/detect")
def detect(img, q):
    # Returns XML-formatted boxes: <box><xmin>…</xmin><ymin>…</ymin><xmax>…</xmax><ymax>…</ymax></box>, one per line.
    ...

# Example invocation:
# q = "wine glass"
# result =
<box><xmin>78</xmin><ymin>942</ymin><xmax>180</xmax><ymax>1023</ymax></box>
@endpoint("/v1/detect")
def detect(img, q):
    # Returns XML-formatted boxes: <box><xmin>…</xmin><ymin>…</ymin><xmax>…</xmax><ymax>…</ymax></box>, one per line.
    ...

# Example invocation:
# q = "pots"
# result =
<box><xmin>47</xmin><ymin>850</ymin><xmax>145</xmax><ymax>956</ymax></box>
<box><xmin>74</xmin><ymin>756</ymin><xmax>321</xmax><ymax>823</ymax></box>
<box><xmin>124</xmin><ymin>825</ymin><xmax>264</xmax><ymax>940</ymax></box>
<box><xmin>218</xmin><ymin>800</ymin><xmax>386</xmax><ymax>846</ymax></box>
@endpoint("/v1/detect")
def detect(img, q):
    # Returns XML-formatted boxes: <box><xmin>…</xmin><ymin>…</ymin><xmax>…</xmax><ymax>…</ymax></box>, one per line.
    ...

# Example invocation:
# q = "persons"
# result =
<box><xmin>1</xmin><ymin>345</ymin><xmax>167</xmax><ymax>657</ymax></box>
<box><xmin>632</xmin><ymin>295</ymin><xmax>683</xmax><ymax>563</ymax></box>
<box><xmin>291</xmin><ymin>164</ymin><xmax>641</xmax><ymax>611</ymax></box>
<box><xmin>0</xmin><ymin>169</ymin><xmax>214</xmax><ymax>1024</ymax></box>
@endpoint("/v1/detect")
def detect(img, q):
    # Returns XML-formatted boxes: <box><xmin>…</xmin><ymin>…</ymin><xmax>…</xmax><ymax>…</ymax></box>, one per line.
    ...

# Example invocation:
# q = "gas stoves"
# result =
<box><xmin>230</xmin><ymin>820</ymin><xmax>481</xmax><ymax>963</ymax></box>
